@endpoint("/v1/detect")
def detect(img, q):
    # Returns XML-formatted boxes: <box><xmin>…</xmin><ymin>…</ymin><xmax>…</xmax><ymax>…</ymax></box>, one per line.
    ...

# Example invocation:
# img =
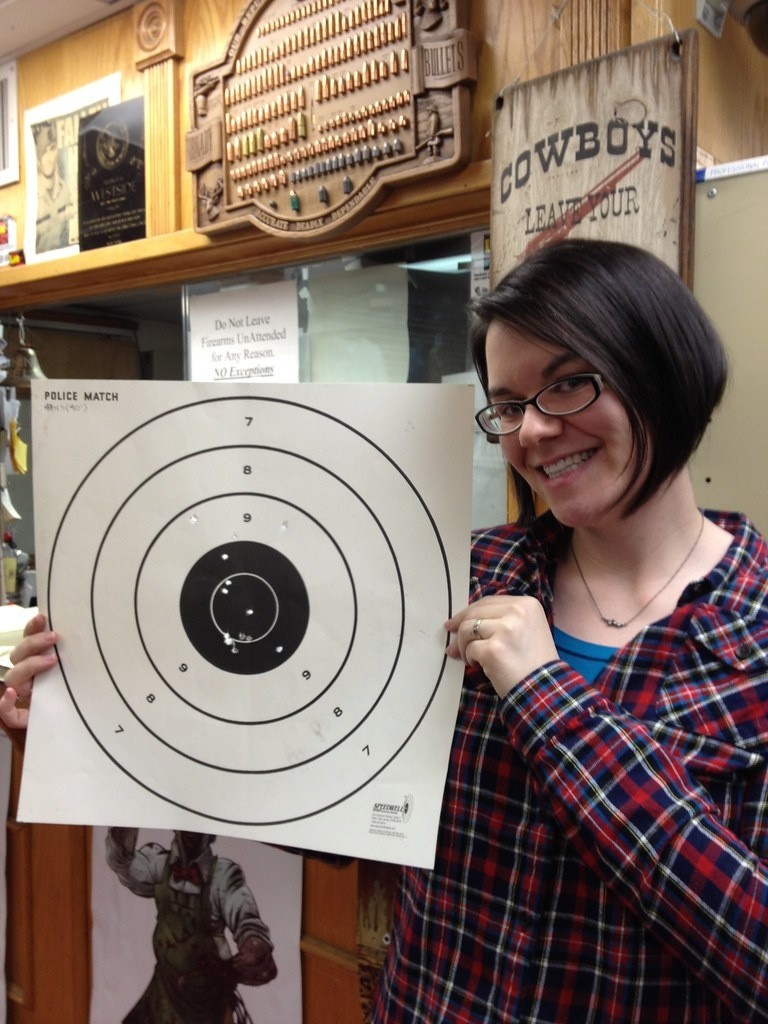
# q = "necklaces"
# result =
<box><xmin>570</xmin><ymin>507</ymin><xmax>704</xmax><ymax>628</ymax></box>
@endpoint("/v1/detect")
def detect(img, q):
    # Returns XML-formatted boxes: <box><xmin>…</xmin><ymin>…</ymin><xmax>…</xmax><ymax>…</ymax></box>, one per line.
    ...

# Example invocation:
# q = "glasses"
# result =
<box><xmin>475</xmin><ymin>374</ymin><xmax>604</xmax><ymax>435</ymax></box>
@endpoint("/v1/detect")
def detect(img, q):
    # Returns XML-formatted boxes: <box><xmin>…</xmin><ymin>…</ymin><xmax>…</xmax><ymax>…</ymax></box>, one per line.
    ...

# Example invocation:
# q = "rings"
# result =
<box><xmin>473</xmin><ymin>618</ymin><xmax>483</xmax><ymax>640</ymax></box>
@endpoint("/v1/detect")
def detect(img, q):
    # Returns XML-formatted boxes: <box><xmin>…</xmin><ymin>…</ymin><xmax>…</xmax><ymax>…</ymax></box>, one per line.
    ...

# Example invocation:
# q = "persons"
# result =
<box><xmin>0</xmin><ymin>239</ymin><xmax>768</xmax><ymax>1024</ymax></box>
